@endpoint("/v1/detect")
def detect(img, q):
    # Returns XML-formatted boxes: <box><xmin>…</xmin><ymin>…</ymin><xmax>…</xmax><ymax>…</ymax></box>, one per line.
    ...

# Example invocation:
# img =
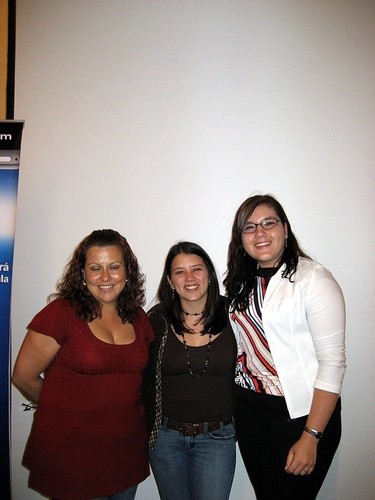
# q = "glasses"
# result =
<box><xmin>242</xmin><ymin>218</ymin><xmax>279</xmax><ymax>234</ymax></box>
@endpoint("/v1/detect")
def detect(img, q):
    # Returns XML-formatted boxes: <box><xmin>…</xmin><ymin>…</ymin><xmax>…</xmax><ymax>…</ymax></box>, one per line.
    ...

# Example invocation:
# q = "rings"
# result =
<box><xmin>303</xmin><ymin>469</ymin><xmax>308</xmax><ymax>473</ymax></box>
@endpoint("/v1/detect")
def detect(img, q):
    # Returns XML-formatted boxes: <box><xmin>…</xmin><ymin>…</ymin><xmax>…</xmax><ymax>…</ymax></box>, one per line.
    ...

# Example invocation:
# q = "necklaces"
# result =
<box><xmin>181</xmin><ymin>310</ymin><xmax>212</xmax><ymax>380</ymax></box>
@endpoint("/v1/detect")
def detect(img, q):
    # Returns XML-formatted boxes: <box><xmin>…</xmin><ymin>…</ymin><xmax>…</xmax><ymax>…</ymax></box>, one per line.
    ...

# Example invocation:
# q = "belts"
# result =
<box><xmin>160</xmin><ymin>418</ymin><xmax>232</xmax><ymax>435</ymax></box>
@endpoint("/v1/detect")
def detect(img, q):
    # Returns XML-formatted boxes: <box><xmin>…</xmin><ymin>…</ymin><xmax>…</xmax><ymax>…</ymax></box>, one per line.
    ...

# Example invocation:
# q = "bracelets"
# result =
<box><xmin>304</xmin><ymin>426</ymin><xmax>323</xmax><ymax>439</ymax></box>
<box><xmin>21</xmin><ymin>401</ymin><xmax>38</xmax><ymax>411</ymax></box>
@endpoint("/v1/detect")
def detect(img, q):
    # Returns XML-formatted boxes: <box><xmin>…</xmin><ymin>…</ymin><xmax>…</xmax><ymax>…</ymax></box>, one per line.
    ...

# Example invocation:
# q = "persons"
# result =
<box><xmin>12</xmin><ymin>229</ymin><xmax>155</xmax><ymax>500</ymax></box>
<box><xmin>146</xmin><ymin>242</ymin><xmax>236</xmax><ymax>500</ymax></box>
<box><xmin>223</xmin><ymin>195</ymin><xmax>347</xmax><ymax>500</ymax></box>
